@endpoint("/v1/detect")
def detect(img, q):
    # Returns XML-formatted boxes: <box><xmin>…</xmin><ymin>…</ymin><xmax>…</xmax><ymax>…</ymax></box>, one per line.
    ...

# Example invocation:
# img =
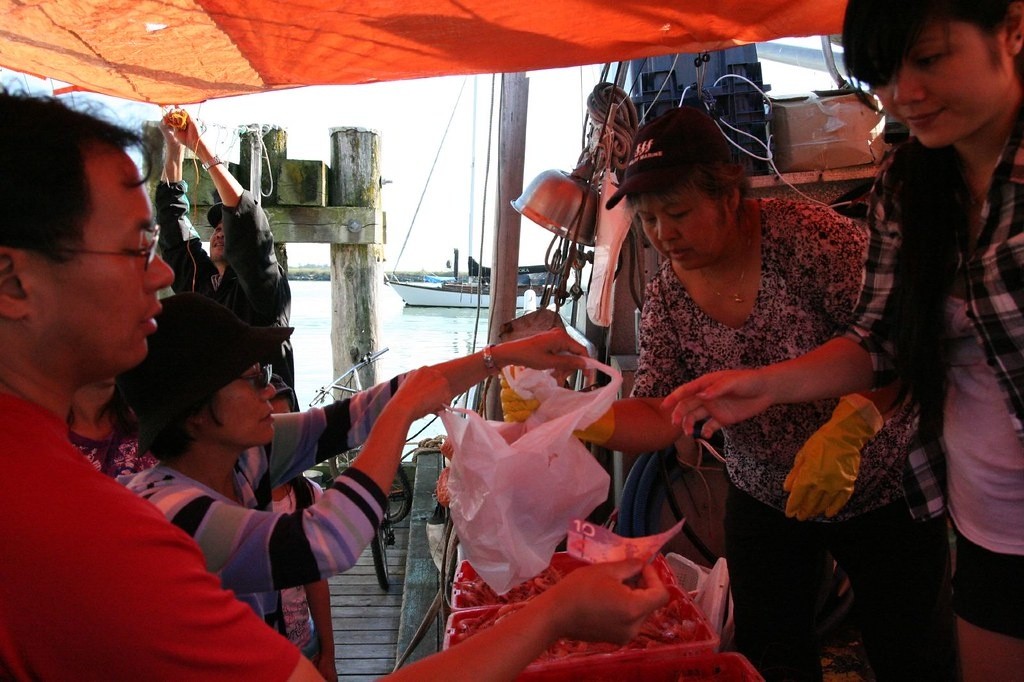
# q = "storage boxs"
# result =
<box><xmin>766</xmin><ymin>81</ymin><xmax>875</xmax><ymax>171</ymax></box>
<box><xmin>637</xmin><ymin>89</ymin><xmax>770</xmax><ymax>176</ymax></box>
<box><xmin>622</xmin><ymin>42</ymin><xmax>768</xmax><ymax>104</ymax></box>
<box><xmin>439</xmin><ymin>550</ymin><xmax>766</xmax><ymax>682</ymax></box>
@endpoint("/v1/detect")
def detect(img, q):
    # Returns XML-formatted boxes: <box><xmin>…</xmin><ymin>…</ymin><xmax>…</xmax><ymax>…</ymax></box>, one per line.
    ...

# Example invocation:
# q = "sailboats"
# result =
<box><xmin>385</xmin><ymin>74</ymin><xmax>575</xmax><ymax>311</ymax></box>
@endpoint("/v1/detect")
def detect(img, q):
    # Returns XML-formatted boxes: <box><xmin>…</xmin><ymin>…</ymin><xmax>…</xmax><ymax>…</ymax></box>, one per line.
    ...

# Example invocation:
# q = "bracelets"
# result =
<box><xmin>482</xmin><ymin>344</ymin><xmax>502</xmax><ymax>376</ymax></box>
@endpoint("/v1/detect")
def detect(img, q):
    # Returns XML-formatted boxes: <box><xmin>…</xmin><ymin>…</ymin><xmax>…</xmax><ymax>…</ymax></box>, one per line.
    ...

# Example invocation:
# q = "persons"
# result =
<box><xmin>499</xmin><ymin>106</ymin><xmax>966</xmax><ymax>682</ymax></box>
<box><xmin>658</xmin><ymin>0</ymin><xmax>1024</xmax><ymax>682</ymax></box>
<box><xmin>0</xmin><ymin>83</ymin><xmax>663</xmax><ymax>682</ymax></box>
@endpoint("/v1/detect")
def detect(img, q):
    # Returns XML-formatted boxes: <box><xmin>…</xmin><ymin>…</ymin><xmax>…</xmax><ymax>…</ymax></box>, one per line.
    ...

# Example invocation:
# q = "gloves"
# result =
<box><xmin>784</xmin><ymin>392</ymin><xmax>884</xmax><ymax>520</ymax></box>
<box><xmin>501</xmin><ymin>366</ymin><xmax>616</xmax><ymax>444</ymax></box>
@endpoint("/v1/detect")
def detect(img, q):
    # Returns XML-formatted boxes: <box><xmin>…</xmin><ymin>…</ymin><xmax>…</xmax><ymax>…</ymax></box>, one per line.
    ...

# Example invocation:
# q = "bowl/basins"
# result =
<box><xmin>509</xmin><ymin>169</ymin><xmax>598</xmax><ymax>247</ymax></box>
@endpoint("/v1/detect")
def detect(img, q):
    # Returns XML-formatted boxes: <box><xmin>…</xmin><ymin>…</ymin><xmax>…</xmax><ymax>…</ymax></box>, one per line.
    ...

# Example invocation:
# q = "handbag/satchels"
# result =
<box><xmin>437</xmin><ymin>353</ymin><xmax>623</xmax><ymax>595</ymax></box>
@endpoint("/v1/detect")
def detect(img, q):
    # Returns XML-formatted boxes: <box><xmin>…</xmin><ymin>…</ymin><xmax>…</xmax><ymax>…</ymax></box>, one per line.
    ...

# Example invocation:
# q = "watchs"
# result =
<box><xmin>201</xmin><ymin>154</ymin><xmax>225</xmax><ymax>172</ymax></box>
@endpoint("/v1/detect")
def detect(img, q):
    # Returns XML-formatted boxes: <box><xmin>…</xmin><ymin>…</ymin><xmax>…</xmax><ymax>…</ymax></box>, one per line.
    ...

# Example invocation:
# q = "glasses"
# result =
<box><xmin>240</xmin><ymin>362</ymin><xmax>275</xmax><ymax>391</ymax></box>
<box><xmin>51</xmin><ymin>221</ymin><xmax>160</xmax><ymax>271</ymax></box>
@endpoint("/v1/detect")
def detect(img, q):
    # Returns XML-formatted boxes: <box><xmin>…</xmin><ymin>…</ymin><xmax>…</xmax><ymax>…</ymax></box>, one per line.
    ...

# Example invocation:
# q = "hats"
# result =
<box><xmin>607</xmin><ymin>107</ymin><xmax>729</xmax><ymax>210</ymax></box>
<box><xmin>135</xmin><ymin>292</ymin><xmax>295</xmax><ymax>404</ymax></box>
<box><xmin>206</xmin><ymin>201</ymin><xmax>224</xmax><ymax>228</ymax></box>
